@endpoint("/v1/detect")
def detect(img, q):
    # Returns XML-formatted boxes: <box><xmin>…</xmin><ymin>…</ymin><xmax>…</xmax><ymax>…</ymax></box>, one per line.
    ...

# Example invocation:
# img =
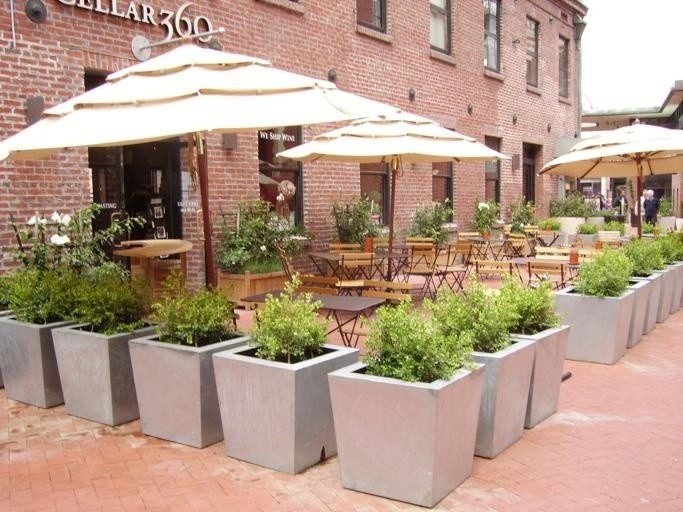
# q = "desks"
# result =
<box><xmin>113</xmin><ymin>239</ymin><xmax>193</xmax><ymax>298</ymax></box>
<box><xmin>240</xmin><ymin>291</ymin><xmax>386</xmax><ymax>349</ymax></box>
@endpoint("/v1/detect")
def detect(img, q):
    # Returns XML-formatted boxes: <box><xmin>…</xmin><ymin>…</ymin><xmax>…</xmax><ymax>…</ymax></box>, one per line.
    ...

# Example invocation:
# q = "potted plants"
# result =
<box><xmin>217</xmin><ymin>202</ymin><xmax>316</xmax><ymax>310</ymax></box>
<box><xmin>536</xmin><ymin>190</ymin><xmax>657</xmax><ymax>245</ymax></box>
<box><xmin>553</xmin><ymin>231</ymin><xmax>682</xmax><ymax>363</ymax></box>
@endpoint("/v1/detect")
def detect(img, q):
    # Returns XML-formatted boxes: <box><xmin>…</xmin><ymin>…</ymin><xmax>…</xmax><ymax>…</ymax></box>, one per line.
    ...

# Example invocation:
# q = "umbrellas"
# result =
<box><xmin>537</xmin><ymin>115</ymin><xmax>683</xmax><ymax>240</ymax></box>
<box><xmin>0</xmin><ymin>34</ymin><xmax>403</xmax><ymax>295</ymax></box>
<box><xmin>275</xmin><ymin>109</ymin><xmax>510</xmax><ymax>296</ymax></box>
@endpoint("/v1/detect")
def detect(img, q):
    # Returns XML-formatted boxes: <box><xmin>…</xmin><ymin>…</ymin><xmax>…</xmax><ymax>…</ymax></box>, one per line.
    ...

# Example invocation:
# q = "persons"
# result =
<box><xmin>613</xmin><ymin>190</ymin><xmax>628</xmax><ymax>216</ymax></box>
<box><xmin>643</xmin><ymin>189</ymin><xmax>658</xmax><ymax>227</ymax></box>
<box><xmin>634</xmin><ymin>189</ymin><xmax>648</xmax><ymax>222</ymax></box>
